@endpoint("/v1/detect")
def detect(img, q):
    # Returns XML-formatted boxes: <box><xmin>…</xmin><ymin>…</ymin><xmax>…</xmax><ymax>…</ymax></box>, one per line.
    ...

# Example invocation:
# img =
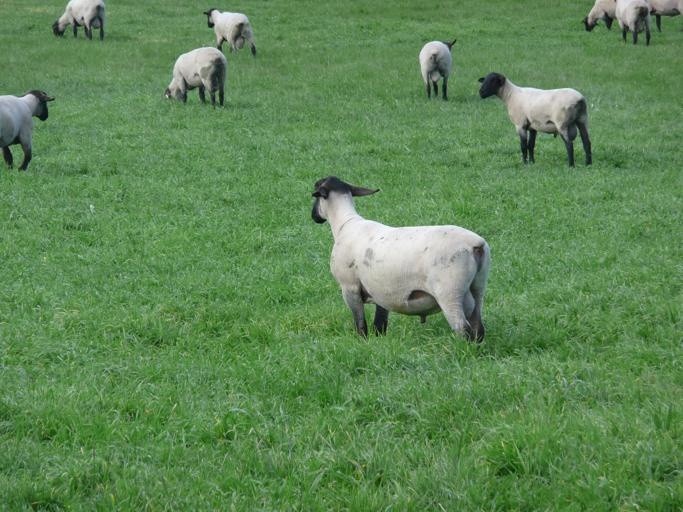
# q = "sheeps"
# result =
<box><xmin>581</xmin><ymin>0</ymin><xmax>617</xmax><ymax>31</ymax></box>
<box><xmin>0</xmin><ymin>88</ymin><xmax>55</xmax><ymax>172</ymax></box>
<box><xmin>311</xmin><ymin>175</ymin><xmax>490</xmax><ymax>348</ymax></box>
<box><xmin>417</xmin><ymin>38</ymin><xmax>458</xmax><ymax>101</ymax></box>
<box><xmin>476</xmin><ymin>72</ymin><xmax>593</xmax><ymax>168</ymax></box>
<box><xmin>163</xmin><ymin>46</ymin><xmax>229</xmax><ymax>107</ymax></box>
<box><xmin>202</xmin><ymin>8</ymin><xmax>258</xmax><ymax>58</ymax></box>
<box><xmin>50</xmin><ymin>0</ymin><xmax>107</xmax><ymax>41</ymax></box>
<box><xmin>649</xmin><ymin>1</ymin><xmax>683</xmax><ymax>32</ymax></box>
<box><xmin>612</xmin><ymin>0</ymin><xmax>655</xmax><ymax>46</ymax></box>
